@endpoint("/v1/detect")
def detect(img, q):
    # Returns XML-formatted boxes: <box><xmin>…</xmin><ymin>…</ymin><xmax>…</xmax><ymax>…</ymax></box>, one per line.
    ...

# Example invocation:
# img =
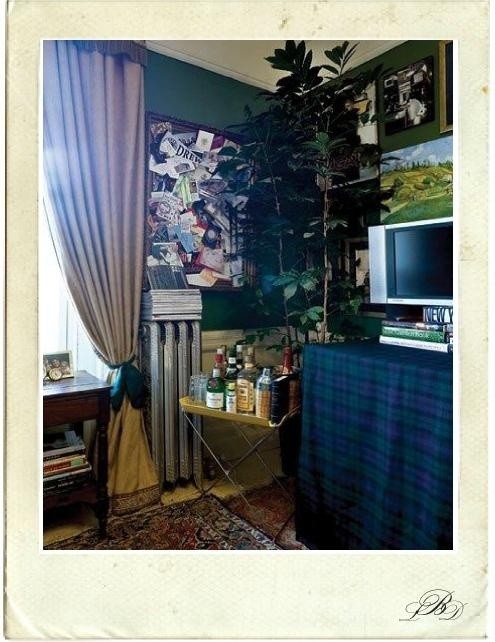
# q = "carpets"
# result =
<box><xmin>44</xmin><ymin>493</ymin><xmax>285</xmax><ymax>549</ymax></box>
<box><xmin>222</xmin><ymin>475</ymin><xmax>310</xmax><ymax>549</ymax></box>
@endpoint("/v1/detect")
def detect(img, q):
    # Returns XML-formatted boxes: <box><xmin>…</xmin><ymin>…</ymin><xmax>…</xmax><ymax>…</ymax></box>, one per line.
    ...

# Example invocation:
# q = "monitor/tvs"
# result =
<box><xmin>367</xmin><ymin>216</ymin><xmax>453</xmax><ymax>308</ymax></box>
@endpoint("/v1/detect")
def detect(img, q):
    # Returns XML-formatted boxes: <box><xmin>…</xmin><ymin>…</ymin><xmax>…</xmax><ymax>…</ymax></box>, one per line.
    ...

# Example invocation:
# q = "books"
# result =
<box><xmin>270</xmin><ymin>375</ymin><xmax>289</xmax><ymax>425</ymax></box>
<box><xmin>43</xmin><ymin>428</ymin><xmax>92</xmax><ymax>497</ymax></box>
<box><xmin>378</xmin><ymin>319</ymin><xmax>453</xmax><ymax>354</ymax></box>
<box><xmin>139</xmin><ymin>288</ymin><xmax>203</xmax><ymax>322</ymax></box>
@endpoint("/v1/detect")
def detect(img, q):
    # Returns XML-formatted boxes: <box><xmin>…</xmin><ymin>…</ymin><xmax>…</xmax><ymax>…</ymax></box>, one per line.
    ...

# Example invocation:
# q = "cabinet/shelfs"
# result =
<box><xmin>43</xmin><ymin>370</ymin><xmax>114</xmax><ymax>540</ymax></box>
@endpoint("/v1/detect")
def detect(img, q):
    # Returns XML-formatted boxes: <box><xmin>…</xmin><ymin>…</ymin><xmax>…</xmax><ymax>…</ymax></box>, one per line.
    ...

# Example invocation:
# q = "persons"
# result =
<box><xmin>43</xmin><ymin>357</ymin><xmax>69</xmax><ymax>376</ymax></box>
<box><xmin>159</xmin><ymin>245</ymin><xmax>172</xmax><ymax>264</ymax></box>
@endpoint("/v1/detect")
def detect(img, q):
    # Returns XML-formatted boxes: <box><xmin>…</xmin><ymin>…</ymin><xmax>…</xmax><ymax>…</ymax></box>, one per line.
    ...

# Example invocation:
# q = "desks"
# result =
<box><xmin>295</xmin><ymin>339</ymin><xmax>453</xmax><ymax>549</ymax></box>
<box><xmin>179</xmin><ymin>396</ymin><xmax>301</xmax><ymax>550</ymax></box>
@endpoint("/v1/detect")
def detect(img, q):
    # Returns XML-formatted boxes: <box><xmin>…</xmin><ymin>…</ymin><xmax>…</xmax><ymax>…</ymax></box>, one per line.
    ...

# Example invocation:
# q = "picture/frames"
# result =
<box><xmin>344</xmin><ymin>237</ymin><xmax>387</xmax><ymax>319</ymax></box>
<box><xmin>438</xmin><ymin>40</ymin><xmax>453</xmax><ymax>134</ymax></box>
<box><xmin>141</xmin><ymin>111</ymin><xmax>260</xmax><ymax>293</ymax></box>
<box><xmin>42</xmin><ymin>350</ymin><xmax>75</xmax><ymax>381</ymax></box>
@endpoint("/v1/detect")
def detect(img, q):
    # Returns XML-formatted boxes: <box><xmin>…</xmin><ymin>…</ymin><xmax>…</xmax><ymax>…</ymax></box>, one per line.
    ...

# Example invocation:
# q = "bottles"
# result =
<box><xmin>206</xmin><ymin>343</ymin><xmax>297</xmax><ymax>424</ymax></box>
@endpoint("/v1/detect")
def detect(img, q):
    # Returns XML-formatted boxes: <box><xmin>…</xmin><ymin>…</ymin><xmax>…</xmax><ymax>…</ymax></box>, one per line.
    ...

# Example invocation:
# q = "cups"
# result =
<box><xmin>188</xmin><ymin>371</ymin><xmax>211</xmax><ymax>404</ymax></box>
